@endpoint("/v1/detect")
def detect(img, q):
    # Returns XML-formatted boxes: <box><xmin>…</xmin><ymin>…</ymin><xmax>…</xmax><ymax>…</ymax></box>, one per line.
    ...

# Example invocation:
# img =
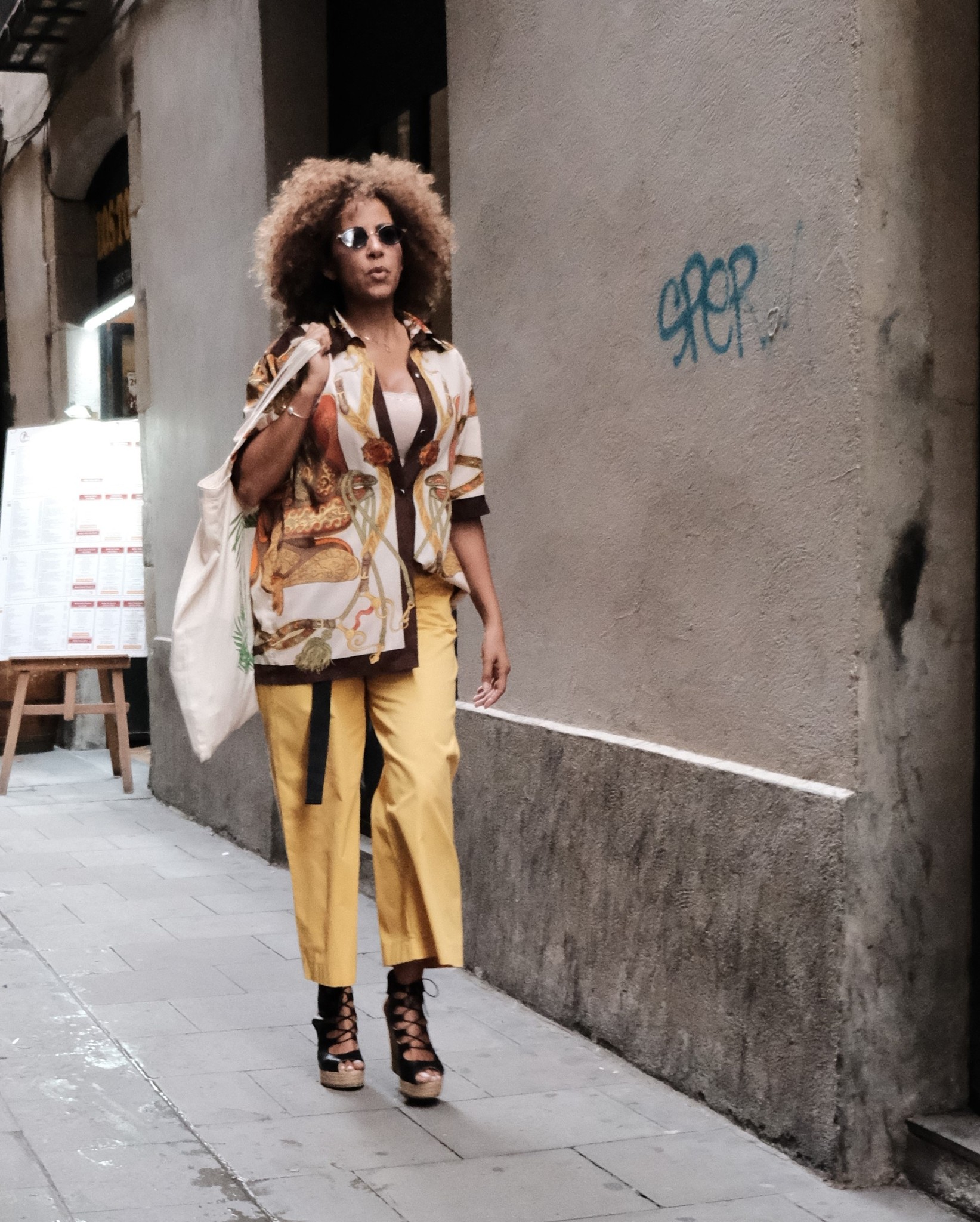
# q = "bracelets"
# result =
<box><xmin>284</xmin><ymin>404</ymin><xmax>311</xmax><ymax>419</ymax></box>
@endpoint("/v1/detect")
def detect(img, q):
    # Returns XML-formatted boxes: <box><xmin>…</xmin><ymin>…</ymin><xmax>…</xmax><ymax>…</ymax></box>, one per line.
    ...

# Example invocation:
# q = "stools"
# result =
<box><xmin>0</xmin><ymin>653</ymin><xmax>134</xmax><ymax>796</ymax></box>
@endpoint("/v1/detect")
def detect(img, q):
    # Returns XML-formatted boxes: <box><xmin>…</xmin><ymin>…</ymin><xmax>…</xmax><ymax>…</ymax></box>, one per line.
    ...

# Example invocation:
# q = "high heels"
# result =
<box><xmin>384</xmin><ymin>969</ymin><xmax>445</xmax><ymax>1098</ymax></box>
<box><xmin>312</xmin><ymin>985</ymin><xmax>365</xmax><ymax>1090</ymax></box>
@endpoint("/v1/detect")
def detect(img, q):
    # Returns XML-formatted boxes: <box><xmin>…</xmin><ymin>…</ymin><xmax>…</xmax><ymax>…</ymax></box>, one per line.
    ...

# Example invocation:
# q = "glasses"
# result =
<box><xmin>335</xmin><ymin>224</ymin><xmax>408</xmax><ymax>248</ymax></box>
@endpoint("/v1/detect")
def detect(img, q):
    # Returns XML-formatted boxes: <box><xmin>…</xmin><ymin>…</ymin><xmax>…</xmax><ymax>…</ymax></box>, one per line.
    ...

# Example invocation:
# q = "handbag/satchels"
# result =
<box><xmin>169</xmin><ymin>339</ymin><xmax>322</xmax><ymax>766</ymax></box>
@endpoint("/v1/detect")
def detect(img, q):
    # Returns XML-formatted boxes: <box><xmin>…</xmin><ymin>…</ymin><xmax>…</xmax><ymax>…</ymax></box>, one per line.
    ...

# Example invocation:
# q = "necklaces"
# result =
<box><xmin>351</xmin><ymin>312</ymin><xmax>392</xmax><ymax>354</ymax></box>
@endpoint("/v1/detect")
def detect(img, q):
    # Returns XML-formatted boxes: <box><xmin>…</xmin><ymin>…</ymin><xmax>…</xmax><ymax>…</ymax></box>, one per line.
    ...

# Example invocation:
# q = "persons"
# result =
<box><xmin>230</xmin><ymin>154</ymin><xmax>512</xmax><ymax>1104</ymax></box>
